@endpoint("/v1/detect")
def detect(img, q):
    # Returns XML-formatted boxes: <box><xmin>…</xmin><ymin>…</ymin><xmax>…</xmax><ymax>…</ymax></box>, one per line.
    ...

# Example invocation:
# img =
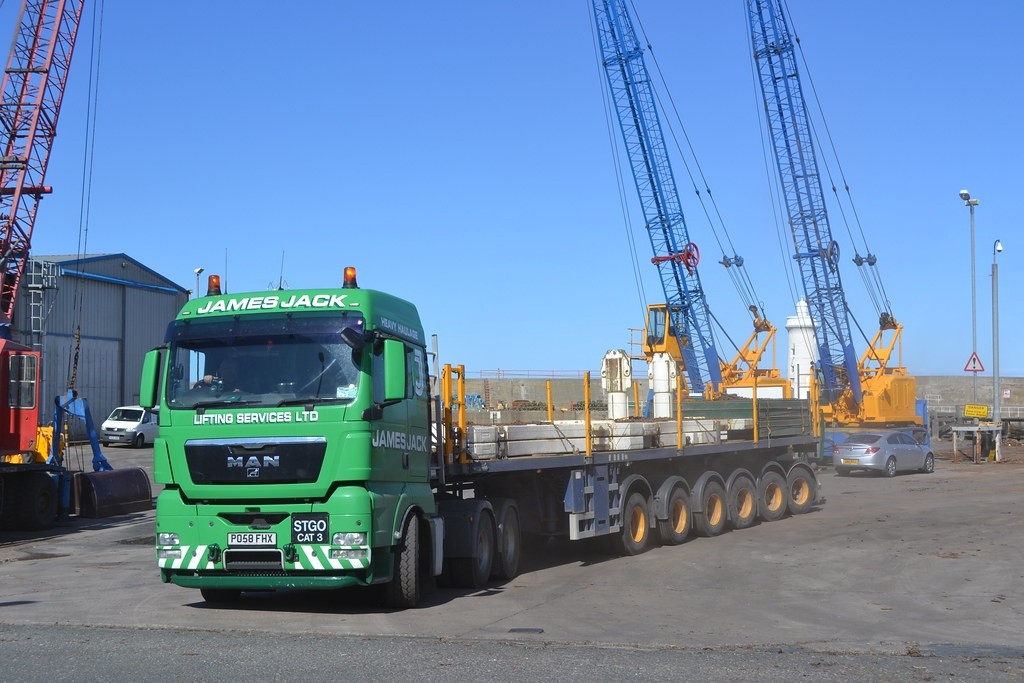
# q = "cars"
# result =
<box><xmin>832</xmin><ymin>430</ymin><xmax>935</xmax><ymax>477</ymax></box>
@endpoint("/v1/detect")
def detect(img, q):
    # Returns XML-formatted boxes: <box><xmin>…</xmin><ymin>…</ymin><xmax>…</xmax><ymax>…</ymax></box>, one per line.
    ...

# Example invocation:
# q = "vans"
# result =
<box><xmin>100</xmin><ymin>404</ymin><xmax>158</xmax><ymax>448</ymax></box>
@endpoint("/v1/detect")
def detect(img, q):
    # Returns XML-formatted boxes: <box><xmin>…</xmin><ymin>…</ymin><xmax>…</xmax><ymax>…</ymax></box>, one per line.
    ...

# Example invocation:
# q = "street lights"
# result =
<box><xmin>992</xmin><ymin>240</ymin><xmax>1004</xmax><ymax>460</ymax></box>
<box><xmin>959</xmin><ymin>190</ymin><xmax>981</xmax><ymax>463</ymax></box>
<box><xmin>194</xmin><ymin>268</ymin><xmax>204</xmax><ymax>384</ymax></box>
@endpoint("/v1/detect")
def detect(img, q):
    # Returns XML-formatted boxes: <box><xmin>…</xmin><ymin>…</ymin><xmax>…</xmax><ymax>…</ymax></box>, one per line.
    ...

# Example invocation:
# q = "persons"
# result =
<box><xmin>201</xmin><ymin>357</ymin><xmax>229</xmax><ymax>394</ymax></box>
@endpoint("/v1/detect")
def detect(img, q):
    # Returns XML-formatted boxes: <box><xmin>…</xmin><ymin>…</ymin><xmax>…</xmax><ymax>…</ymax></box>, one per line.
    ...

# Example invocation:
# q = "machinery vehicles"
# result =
<box><xmin>5</xmin><ymin>390</ymin><xmax>152</xmax><ymax>517</ymax></box>
<box><xmin>0</xmin><ymin>0</ymin><xmax>87</xmax><ymax>530</ymax></box>
<box><xmin>590</xmin><ymin>0</ymin><xmax>924</xmax><ymax>457</ymax></box>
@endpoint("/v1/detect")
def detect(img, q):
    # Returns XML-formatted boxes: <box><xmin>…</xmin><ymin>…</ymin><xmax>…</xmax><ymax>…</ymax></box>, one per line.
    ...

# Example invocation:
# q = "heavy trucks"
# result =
<box><xmin>139</xmin><ymin>266</ymin><xmax>825</xmax><ymax>607</ymax></box>
